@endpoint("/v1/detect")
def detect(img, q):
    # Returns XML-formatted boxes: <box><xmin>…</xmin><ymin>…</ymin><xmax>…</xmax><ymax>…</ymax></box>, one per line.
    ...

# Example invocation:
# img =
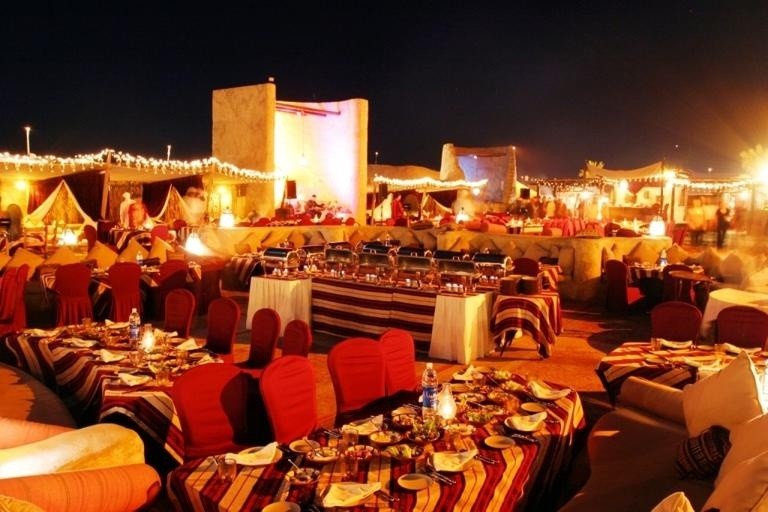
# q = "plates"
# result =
<box><xmin>484</xmin><ymin>436</ymin><xmax>515</xmax><ymax>449</ymax></box>
<box><xmin>189</xmin><ymin>352</ymin><xmax>209</xmax><ymax>359</ymax></box>
<box><xmin>284</xmin><ymin>468</ymin><xmax>320</xmax><ymax>486</ymax></box>
<box><xmin>482</xmin><ymin>405</ymin><xmax>504</xmax><ymax>415</ymax></box>
<box><xmin>168</xmin><ymin>337</ymin><xmax>185</xmax><ymax>343</ymax></box>
<box><xmin>92</xmin><ymin>350</ymin><xmax>111</xmax><ymax>356</ymax></box>
<box><xmin>391</xmin><ymin>409</ymin><xmax>417</xmax><ymax>417</ymax></box>
<box><xmin>306</xmin><ymin>447</ymin><xmax>340</xmax><ymax>463</ymax></box>
<box><xmin>392</xmin><ymin>415</ymin><xmax>422</xmax><ymax>427</ymax></box>
<box><xmin>459</xmin><ymin>393</ymin><xmax>486</xmax><ymax>402</ymax></box>
<box><xmin>112</xmin><ymin>375</ymin><xmax>152</xmax><ymax>387</ymax></box>
<box><xmin>174</xmin><ymin>343</ymin><xmax>203</xmax><ymax>351</ymax></box>
<box><xmin>521</xmin><ymin>403</ymin><xmax>546</xmax><ymax>413</ymax></box>
<box><xmin>96</xmin><ymin>355</ymin><xmax>127</xmax><ymax>363</ymax></box>
<box><xmin>236</xmin><ymin>447</ymin><xmax>283</xmax><ymax>466</ymax></box>
<box><xmin>426</xmin><ymin>451</ymin><xmax>474</xmax><ymax>473</ymax></box>
<box><xmin>261</xmin><ymin>502</ymin><xmax>301</xmax><ymax>512</ymax></box>
<box><xmin>504</xmin><ymin>416</ymin><xmax>546</xmax><ymax>432</ymax></box>
<box><xmin>444</xmin><ymin>424</ymin><xmax>477</xmax><ymax>436</ymax></box>
<box><xmin>349</xmin><ymin>420</ymin><xmax>388</xmax><ymax>436</ymax></box>
<box><xmin>319</xmin><ymin>482</ymin><xmax>374</xmax><ymax>507</ymax></box>
<box><xmin>344</xmin><ymin>445</ymin><xmax>378</xmax><ymax>460</ymax></box>
<box><xmin>288</xmin><ymin>440</ymin><xmax>320</xmax><ymax>454</ymax></box>
<box><xmin>369</xmin><ymin>431</ymin><xmax>402</xmax><ymax>444</ymax></box>
<box><xmin>499</xmin><ymin>276</ymin><xmax>517</xmax><ymax>297</ymax></box>
<box><xmin>522</xmin><ymin>275</ymin><xmax>543</xmax><ymax>296</ymax></box>
<box><xmin>397</xmin><ymin>473</ymin><xmax>432</xmax><ymax>491</ymax></box>
<box><xmin>405</xmin><ymin>430</ymin><xmax>440</xmax><ymax>442</ymax></box>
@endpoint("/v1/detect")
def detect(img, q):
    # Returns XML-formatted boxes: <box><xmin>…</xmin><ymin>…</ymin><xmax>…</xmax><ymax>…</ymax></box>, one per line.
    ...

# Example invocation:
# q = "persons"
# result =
<box><xmin>685</xmin><ymin>195</ymin><xmax>734</xmax><ymax>249</ymax></box>
<box><xmin>506</xmin><ymin>193</ymin><xmax>572</xmax><ymax>218</ymax></box>
<box><xmin>304</xmin><ymin>194</ymin><xmax>323</xmax><ymax>223</ymax></box>
<box><xmin>392</xmin><ymin>193</ymin><xmax>407</xmax><ymax>227</ymax></box>
<box><xmin>117</xmin><ymin>191</ymin><xmax>150</xmax><ymax>230</ymax></box>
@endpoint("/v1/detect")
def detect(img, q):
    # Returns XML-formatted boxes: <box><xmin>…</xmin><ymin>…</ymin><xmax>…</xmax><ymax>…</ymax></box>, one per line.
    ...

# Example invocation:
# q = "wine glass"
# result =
<box><xmin>148</xmin><ymin>353</ymin><xmax>164</xmax><ymax>385</ymax></box>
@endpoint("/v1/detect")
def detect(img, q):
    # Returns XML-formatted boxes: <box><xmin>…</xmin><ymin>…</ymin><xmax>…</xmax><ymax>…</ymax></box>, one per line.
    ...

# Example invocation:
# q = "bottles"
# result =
<box><xmin>136</xmin><ymin>251</ymin><xmax>143</xmax><ymax>267</ymax></box>
<box><xmin>422</xmin><ymin>363</ymin><xmax>439</xmax><ymax>416</ymax></box>
<box><xmin>660</xmin><ymin>248</ymin><xmax>668</xmax><ymax>267</ymax></box>
<box><xmin>129</xmin><ymin>308</ymin><xmax>140</xmax><ymax>344</ymax></box>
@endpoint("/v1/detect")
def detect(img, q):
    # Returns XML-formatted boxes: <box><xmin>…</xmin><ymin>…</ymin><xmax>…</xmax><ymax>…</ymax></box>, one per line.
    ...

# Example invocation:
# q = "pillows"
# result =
<box><xmin>700</xmin><ymin>451</ymin><xmax>768</xmax><ymax>512</ymax></box>
<box><xmin>651</xmin><ymin>492</ymin><xmax>695</xmax><ymax>512</ymax></box>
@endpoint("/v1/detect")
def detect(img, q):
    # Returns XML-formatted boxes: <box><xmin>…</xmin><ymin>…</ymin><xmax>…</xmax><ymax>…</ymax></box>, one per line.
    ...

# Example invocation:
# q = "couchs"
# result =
<box><xmin>558</xmin><ymin>408</ymin><xmax>768</xmax><ymax>512</ymax></box>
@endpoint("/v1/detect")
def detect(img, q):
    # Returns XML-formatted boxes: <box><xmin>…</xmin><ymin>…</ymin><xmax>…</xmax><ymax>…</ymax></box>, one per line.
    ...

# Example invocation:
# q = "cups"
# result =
<box><xmin>711</xmin><ymin>341</ymin><xmax>728</xmax><ymax>363</ymax></box>
<box><xmin>651</xmin><ymin>337</ymin><xmax>661</xmax><ymax>352</ymax></box>
<box><xmin>176</xmin><ymin>349</ymin><xmax>189</xmax><ymax>366</ymax></box>
<box><xmin>526</xmin><ymin>373</ymin><xmax>539</xmax><ymax>389</ymax></box>
<box><xmin>158</xmin><ymin>334</ymin><xmax>170</xmax><ymax>346</ymax></box>
<box><xmin>270</xmin><ymin>266</ymin><xmax>290</xmax><ymax>278</ymax></box>
<box><xmin>404</xmin><ymin>277</ymin><xmax>417</xmax><ymax>289</ymax></box>
<box><xmin>444</xmin><ymin>281</ymin><xmax>465</xmax><ymax>294</ymax></box>
<box><xmin>365</xmin><ymin>274</ymin><xmax>378</xmax><ymax>285</ymax></box>
<box><xmin>342</xmin><ymin>429</ymin><xmax>359</xmax><ymax>450</ymax></box>
<box><xmin>472</xmin><ymin>373</ymin><xmax>484</xmax><ymax>389</ymax></box>
<box><xmin>218</xmin><ymin>458</ymin><xmax>237</xmax><ymax>482</ymax></box>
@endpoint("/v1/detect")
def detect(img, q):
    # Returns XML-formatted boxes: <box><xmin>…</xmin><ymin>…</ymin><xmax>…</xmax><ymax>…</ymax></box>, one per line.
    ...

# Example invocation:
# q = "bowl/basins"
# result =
<box><xmin>460</xmin><ymin>413</ymin><xmax>493</xmax><ymax>427</ymax></box>
